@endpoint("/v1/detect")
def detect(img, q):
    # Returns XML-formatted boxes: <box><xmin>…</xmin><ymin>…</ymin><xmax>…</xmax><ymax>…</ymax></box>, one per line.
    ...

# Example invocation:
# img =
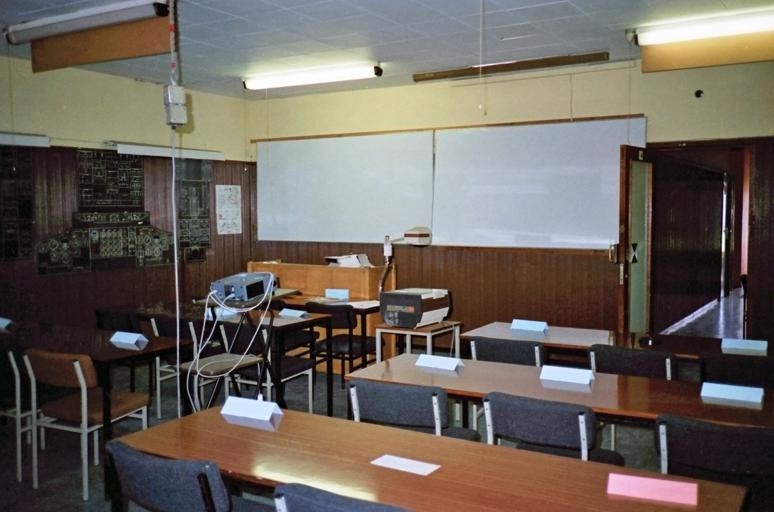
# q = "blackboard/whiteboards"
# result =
<box><xmin>256</xmin><ymin>113</ymin><xmax>649</xmax><ymax>251</ymax></box>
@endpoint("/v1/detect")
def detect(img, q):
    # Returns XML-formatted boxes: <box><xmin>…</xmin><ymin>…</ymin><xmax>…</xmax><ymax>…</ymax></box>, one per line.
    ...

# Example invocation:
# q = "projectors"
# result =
<box><xmin>212</xmin><ymin>272</ymin><xmax>279</xmax><ymax>302</ymax></box>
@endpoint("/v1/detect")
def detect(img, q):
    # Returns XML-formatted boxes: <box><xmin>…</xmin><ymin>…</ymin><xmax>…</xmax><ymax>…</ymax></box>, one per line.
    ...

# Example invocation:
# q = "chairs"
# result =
<box><xmin>470</xmin><ymin>337</ymin><xmax>543</xmax><ymax>368</ymax></box>
<box><xmin>695</xmin><ymin>356</ymin><xmax>774</xmax><ymax>394</ymax></box>
<box><xmin>349</xmin><ymin>379</ymin><xmax>481</xmax><ymax>443</ymax></box>
<box><xmin>654</xmin><ymin>413</ymin><xmax>772</xmax><ymax>509</ymax></box>
<box><xmin>484</xmin><ymin>391</ymin><xmax>626</xmax><ymax>467</ymax></box>
<box><xmin>139</xmin><ymin>312</ymin><xmax>190</xmax><ymax>421</ymax></box>
<box><xmin>590</xmin><ymin>343</ymin><xmax>674</xmax><ymax>379</ymax></box>
<box><xmin>305</xmin><ymin>300</ymin><xmax>385</xmax><ymax>393</ymax></box>
<box><xmin>2</xmin><ymin>327</ymin><xmax>44</xmax><ymax>483</ymax></box>
<box><xmin>23</xmin><ymin>350</ymin><xmax>150</xmax><ymax>502</ymax></box>
<box><xmin>107</xmin><ymin>441</ymin><xmax>228</xmax><ymax>510</ymax></box>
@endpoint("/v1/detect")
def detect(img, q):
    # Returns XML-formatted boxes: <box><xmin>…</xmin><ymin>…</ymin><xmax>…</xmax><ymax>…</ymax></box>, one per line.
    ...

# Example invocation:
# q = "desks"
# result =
<box><xmin>93</xmin><ymin>299</ymin><xmax>334</xmax><ymax>418</ymax></box>
<box><xmin>194</xmin><ymin>290</ymin><xmax>380</xmax><ymax>393</ymax></box>
<box><xmin>459</xmin><ymin>320</ymin><xmax>773</xmax><ymax>361</ymax></box>
<box><xmin>3</xmin><ymin>315</ymin><xmax>194</xmax><ymax>447</ymax></box>
<box><xmin>345</xmin><ymin>353</ymin><xmax>774</xmax><ymax>452</ymax></box>
<box><xmin>375</xmin><ymin>320</ymin><xmax>462</xmax><ymax>364</ymax></box>
<box><xmin>105</xmin><ymin>400</ymin><xmax>746</xmax><ymax>510</ymax></box>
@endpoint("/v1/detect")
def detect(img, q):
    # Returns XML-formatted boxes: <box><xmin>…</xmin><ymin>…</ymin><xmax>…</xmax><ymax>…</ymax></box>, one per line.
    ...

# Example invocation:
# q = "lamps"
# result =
<box><xmin>4</xmin><ymin>0</ymin><xmax>170</xmax><ymax>46</ymax></box>
<box><xmin>241</xmin><ymin>64</ymin><xmax>383</xmax><ymax>91</ymax></box>
<box><xmin>625</xmin><ymin>6</ymin><xmax>773</xmax><ymax>47</ymax></box>
